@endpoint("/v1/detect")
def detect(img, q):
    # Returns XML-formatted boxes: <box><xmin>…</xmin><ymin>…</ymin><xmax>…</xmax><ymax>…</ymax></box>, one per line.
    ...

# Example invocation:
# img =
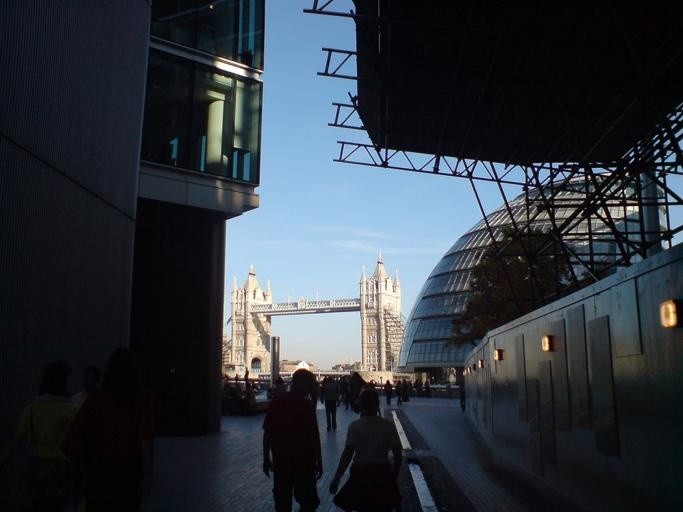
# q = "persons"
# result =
<box><xmin>329</xmin><ymin>387</ymin><xmax>404</xmax><ymax>512</ymax></box>
<box><xmin>1</xmin><ymin>347</ymin><xmax>153</xmax><ymax>512</ymax></box>
<box><xmin>459</xmin><ymin>381</ymin><xmax>466</xmax><ymax>411</ymax></box>
<box><xmin>261</xmin><ymin>369</ymin><xmax>323</xmax><ymax>512</ymax></box>
<box><xmin>220</xmin><ymin>366</ymin><xmax>451</xmax><ymax>431</ymax></box>
